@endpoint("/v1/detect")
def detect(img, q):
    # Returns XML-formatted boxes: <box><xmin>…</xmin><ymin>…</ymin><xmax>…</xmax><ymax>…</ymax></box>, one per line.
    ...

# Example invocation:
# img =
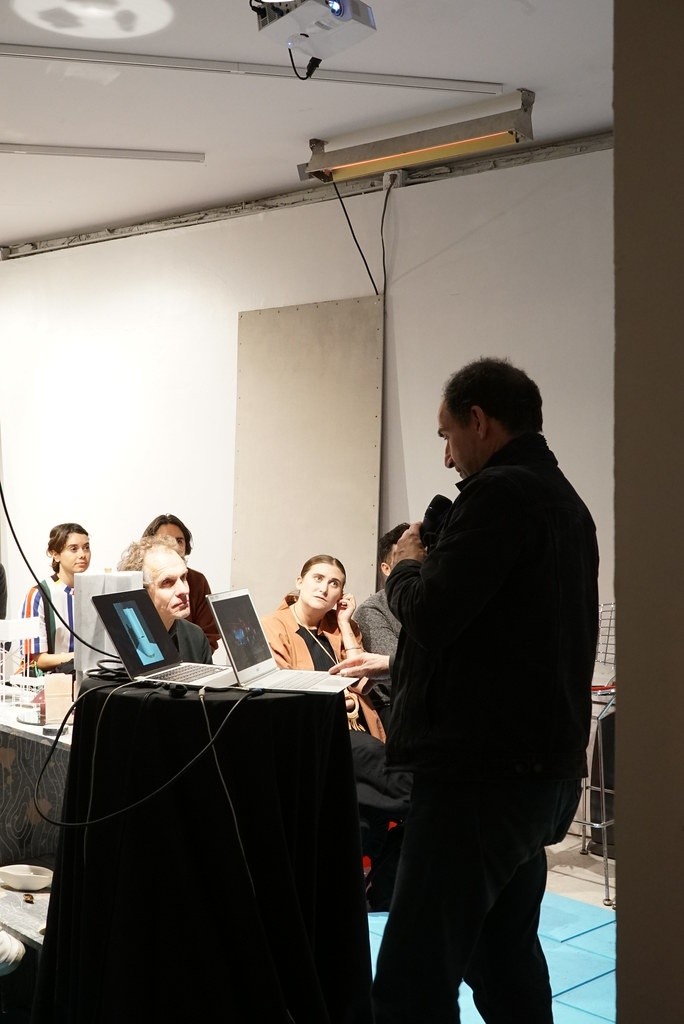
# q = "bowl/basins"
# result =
<box><xmin>0</xmin><ymin>865</ymin><xmax>53</xmax><ymax>891</ymax></box>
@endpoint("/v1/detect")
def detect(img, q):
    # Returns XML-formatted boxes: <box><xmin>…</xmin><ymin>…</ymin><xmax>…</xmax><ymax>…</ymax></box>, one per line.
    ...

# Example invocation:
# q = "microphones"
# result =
<box><xmin>419</xmin><ymin>494</ymin><xmax>452</xmax><ymax>549</ymax></box>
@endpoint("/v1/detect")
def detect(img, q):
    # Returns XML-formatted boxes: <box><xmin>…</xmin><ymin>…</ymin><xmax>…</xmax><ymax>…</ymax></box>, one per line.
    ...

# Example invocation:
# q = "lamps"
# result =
<box><xmin>297</xmin><ymin>88</ymin><xmax>535</xmax><ymax>183</ymax></box>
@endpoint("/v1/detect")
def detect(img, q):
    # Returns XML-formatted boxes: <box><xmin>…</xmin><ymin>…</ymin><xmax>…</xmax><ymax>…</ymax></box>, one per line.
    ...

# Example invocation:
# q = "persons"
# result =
<box><xmin>0</xmin><ymin>562</ymin><xmax>12</xmax><ymax>655</ymax></box>
<box><xmin>15</xmin><ymin>523</ymin><xmax>92</xmax><ymax>676</ymax></box>
<box><xmin>372</xmin><ymin>353</ymin><xmax>600</xmax><ymax>1024</ymax></box>
<box><xmin>86</xmin><ymin>514</ymin><xmax>412</xmax><ymax>913</ymax></box>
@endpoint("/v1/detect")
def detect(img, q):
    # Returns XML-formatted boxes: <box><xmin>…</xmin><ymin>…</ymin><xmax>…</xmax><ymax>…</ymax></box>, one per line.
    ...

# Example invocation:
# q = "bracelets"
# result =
<box><xmin>343</xmin><ymin>646</ymin><xmax>363</xmax><ymax>653</ymax></box>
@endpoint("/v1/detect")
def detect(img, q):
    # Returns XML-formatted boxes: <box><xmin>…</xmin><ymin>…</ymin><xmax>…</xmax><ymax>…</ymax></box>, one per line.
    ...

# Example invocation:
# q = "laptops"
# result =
<box><xmin>91</xmin><ymin>588</ymin><xmax>239</xmax><ymax>692</ymax></box>
<box><xmin>206</xmin><ymin>589</ymin><xmax>360</xmax><ymax>695</ymax></box>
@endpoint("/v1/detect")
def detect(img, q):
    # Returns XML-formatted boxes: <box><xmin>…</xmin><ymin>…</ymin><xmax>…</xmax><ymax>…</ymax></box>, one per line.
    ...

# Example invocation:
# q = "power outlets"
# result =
<box><xmin>383</xmin><ymin>170</ymin><xmax>407</xmax><ymax>191</ymax></box>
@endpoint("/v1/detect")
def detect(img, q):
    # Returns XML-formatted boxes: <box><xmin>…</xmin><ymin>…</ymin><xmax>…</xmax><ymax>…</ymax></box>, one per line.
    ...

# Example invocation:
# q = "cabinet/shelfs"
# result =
<box><xmin>0</xmin><ymin>683</ymin><xmax>71</xmax><ymax>867</ymax></box>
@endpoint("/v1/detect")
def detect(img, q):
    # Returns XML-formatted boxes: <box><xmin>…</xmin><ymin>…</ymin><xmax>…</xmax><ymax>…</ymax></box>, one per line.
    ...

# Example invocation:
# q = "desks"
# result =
<box><xmin>36</xmin><ymin>668</ymin><xmax>372</xmax><ymax>1024</ymax></box>
